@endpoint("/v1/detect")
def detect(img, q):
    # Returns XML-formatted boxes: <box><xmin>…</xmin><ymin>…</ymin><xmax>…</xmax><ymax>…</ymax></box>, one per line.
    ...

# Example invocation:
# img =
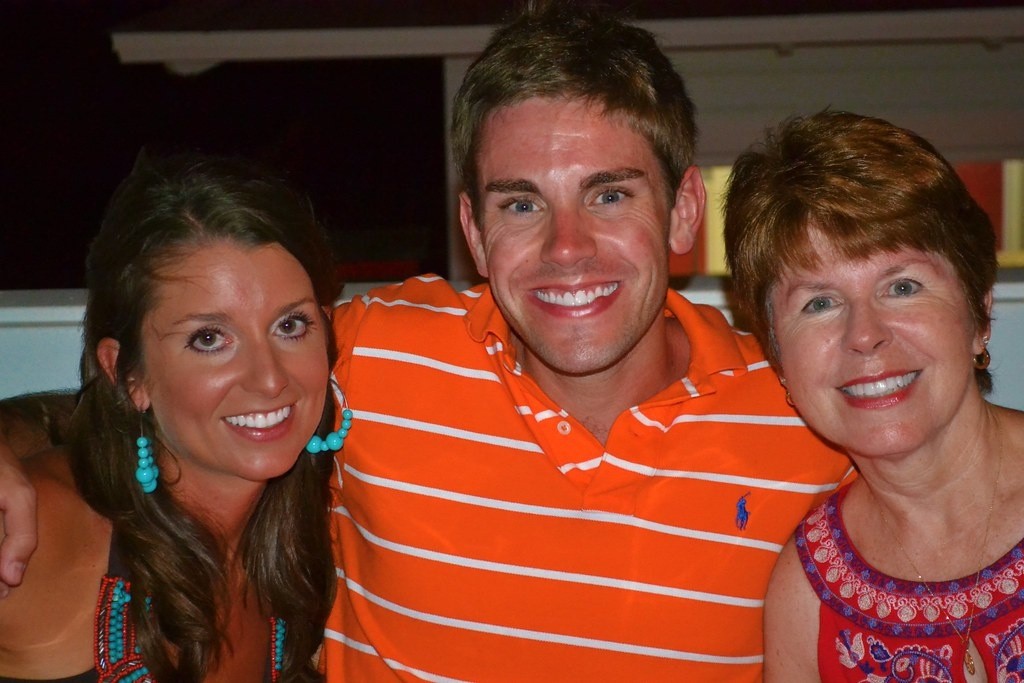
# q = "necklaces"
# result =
<box><xmin>876</xmin><ymin>408</ymin><xmax>1005</xmax><ymax>676</ymax></box>
<box><xmin>92</xmin><ymin>574</ymin><xmax>287</xmax><ymax>682</ymax></box>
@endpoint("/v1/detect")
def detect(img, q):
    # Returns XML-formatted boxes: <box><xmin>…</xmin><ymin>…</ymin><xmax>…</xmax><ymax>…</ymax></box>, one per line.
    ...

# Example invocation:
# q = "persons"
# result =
<box><xmin>724</xmin><ymin>111</ymin><xmax>1023</xmax><ymax>683</ymax></box>
<box><xmin>0</xmin><ymin>146</ymin><xmax>354</xmax><ymax>683</ymax></box>
<box><xmin>0</xmin><ymin>17</ymin><xmax>859</xmax><ymax>683</ymax></box>
